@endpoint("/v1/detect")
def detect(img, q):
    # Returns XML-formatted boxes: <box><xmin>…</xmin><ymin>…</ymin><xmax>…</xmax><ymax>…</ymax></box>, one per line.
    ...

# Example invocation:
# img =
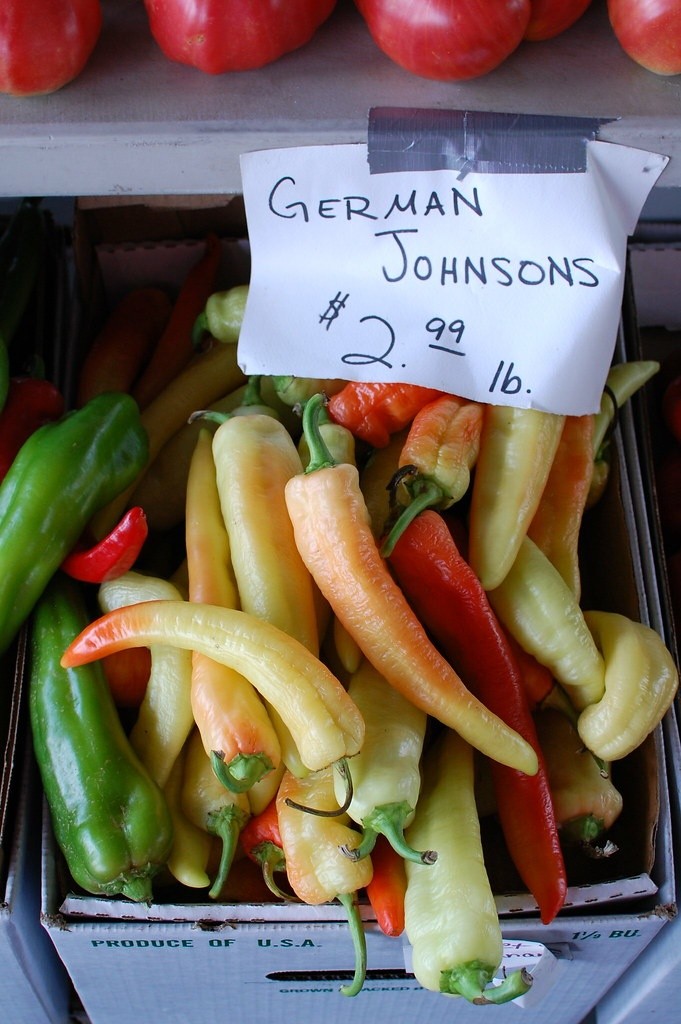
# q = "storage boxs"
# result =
<box><xmin>0</xmin><ymin>197</ymin><xmax>681</xmax><ymax>1024</ymax></box>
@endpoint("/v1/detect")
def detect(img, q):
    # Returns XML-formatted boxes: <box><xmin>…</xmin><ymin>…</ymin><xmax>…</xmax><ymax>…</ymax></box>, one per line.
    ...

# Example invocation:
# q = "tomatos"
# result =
<box><xmin>0</xmin><ymin>0</ymin><xmax>681</xmax><ymax>98</ymax></box>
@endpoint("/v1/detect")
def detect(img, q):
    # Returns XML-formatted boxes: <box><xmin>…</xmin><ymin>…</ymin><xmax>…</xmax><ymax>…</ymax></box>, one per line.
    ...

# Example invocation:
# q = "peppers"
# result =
<box><xmin>0</xmin><ymin>193</ymin><xmax>677</xmax><ymax>1003</ymax></box>
